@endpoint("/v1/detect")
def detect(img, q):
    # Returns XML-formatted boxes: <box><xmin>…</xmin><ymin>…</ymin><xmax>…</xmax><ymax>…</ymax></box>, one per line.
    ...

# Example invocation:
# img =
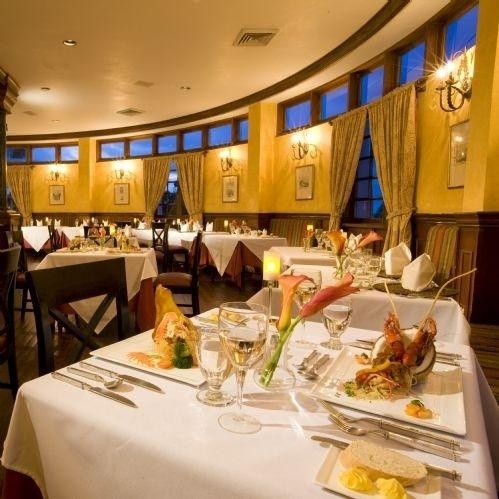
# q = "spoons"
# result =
<box><xmin>292</xmin><ymin>350</ymin><xmax>320</xmax><ymax>371</ymax></box>
<box><xmin>67</xmin><ymin>366</ymin><xmax>124</xmax><ymax>388</ymax></box>
<box><xmin>298</xmin><ymin>354</ymin><xmax>331</xmax><ymax>379</ymax></box>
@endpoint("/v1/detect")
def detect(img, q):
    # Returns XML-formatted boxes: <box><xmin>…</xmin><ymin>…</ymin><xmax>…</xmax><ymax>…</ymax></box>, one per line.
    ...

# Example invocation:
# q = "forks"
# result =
<box><xmin>319</xmin><ymin>399</ymin><xmax>461</xmax><ymax>449</ymax></box>
<box><xmin>328</xmin><ymin>415</ymin><xmax>461</xmax><ymax>462</ymax></box>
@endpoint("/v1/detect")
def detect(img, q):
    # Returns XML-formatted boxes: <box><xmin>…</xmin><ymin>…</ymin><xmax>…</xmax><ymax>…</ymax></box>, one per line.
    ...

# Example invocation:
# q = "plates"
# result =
<box><xmin>89</xmin><ymin>327</ymin><xmax>218</xmax><ymax>387</ymax></box>
<box><xmin>374</xmin><ymin>282</ymin><xmax>458</xmax><ymax>297</ymax></box>
<box><xmin>309</xmin><ymin>345</ymin><xmax>467</xmax><ymax>436</ymax></box>
<box><xmin>311</xmin><ymin>444</ymin><xmax>442</xmax><ymax>499</ymax></box>
<box><xmin>377</xmin><ymin>268</ymin><xmax>402</xmax><ymax>280</ymax></box>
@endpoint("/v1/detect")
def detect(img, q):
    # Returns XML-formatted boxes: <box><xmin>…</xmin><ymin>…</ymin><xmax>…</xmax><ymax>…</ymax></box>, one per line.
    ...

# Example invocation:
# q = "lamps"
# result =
<box><xmin>290</xmin><ymin>130</ymin><xmax>318</xmax><ymax>160</ymax></box>
<box><xmin>432</xmin><ymin>46</ymin><xmax>472</xmax><ymax>113</ymax></box>
<box><xmin>219</xmin><ymin>147</ymin><xmax>239</xmax><ymax>171</ymax></box>
<box><xmin>48</xmin><ymin>162</ymin><xmax>64</xmax><ymax>181</ymax></box>
<box><xmin>114</xmin><ymin>161</ymin><xmax>136</xmax><ymax>179</ymax></box>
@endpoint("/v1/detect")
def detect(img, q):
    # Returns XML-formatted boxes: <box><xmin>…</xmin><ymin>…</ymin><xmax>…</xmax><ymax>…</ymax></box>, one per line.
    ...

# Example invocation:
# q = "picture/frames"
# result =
<box><xmin>222</xmin><ymin>175</ymin><xmax>239</xmax><ymax>203</ymax></box>
<box><xmin>447</xmin><ymin>119</ymin><xmax>469</xmax><ymax>188</ymax></box>
<box><xmin>294</xmin><ymin>164</ymin><xmax>315</xmax><ymax>201</ymax></box>
<box><xmin>49</xmin><ymin>184</ymin><xmax>65</xmax><ymax>205</ymax></box>
<box><xmin>114</xmin><ymin>183</ymin><xmax>130</xmax><ymax>205</ymax></box>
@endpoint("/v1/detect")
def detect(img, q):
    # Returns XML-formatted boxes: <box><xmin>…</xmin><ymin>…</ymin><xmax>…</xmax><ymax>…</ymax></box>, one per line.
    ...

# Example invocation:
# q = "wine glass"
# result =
<box><xmin>321</xmin><ymin>296</ymin><xmax>352</xmax><ymax>349</ymax></box>
<box><xmin>196</xmin><ymin>339</ymin><xmax>235</xmax><ymax>406</ymax></box>
<box><xmin>228</xmin><ymin>222</ymin><xmax>252</xmax><ymax>235</ymax></box>
<box><xmin>178</xmin><ymin>220</ymin><xmax>214</xmax><ymax>231</ymax></box>
<box><xmin>31</xmin><ymin>218</ymin><xmax>60</xmax><ymax>226</ymax></box>
<box><xmin>303</xmin><ymin>229</ymin><xmax>331</xmax><ymax>254</ymax></box>
<box><xmin>218</xmin><ymin>303</ymin><xmax>268</xmax><ymax>435</ymax></box>
<box><xmin>133</xmin><ymin>218</ymin><xmax>167</xmax><ymax>228</ymax></box>
<box><xmin>68</xmin><ymin>232</ymin><xmax>140</xmax><ymax>253</ymax></box>
<box><xmin>292</xmin><ymin>267</ymin><xmax>322</xmax><ymax>351</ymax></box>
<box><xmin>75</xmin><ymin>218</ymin><xmax>108</xmax><ymax>227</ymax></box>
<box><xmin>350</xmin><ymin>246</ymin><xmax>381</xmax><ymax>290</ymax></box>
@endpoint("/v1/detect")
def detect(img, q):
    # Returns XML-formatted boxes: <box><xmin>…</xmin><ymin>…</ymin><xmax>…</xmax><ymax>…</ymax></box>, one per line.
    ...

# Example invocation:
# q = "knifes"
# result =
<box><xmin>349</xmin><ymin>338</ymin><xmax>462</xmax><ymax>367</ymax></box>
<box><xmin>80</xmin><ymin>360</ymin><xmax>161</xmax><ymax>392</ymax></box>
<box><xmin>310</xmin><ymin>435</ymin><xmax>463</xmax><ymax>481</ymax></box>
<box><xmin>51</xmin><ymin>371</ymin><xmax>135</xmax><ymax>408</ymax></box>
